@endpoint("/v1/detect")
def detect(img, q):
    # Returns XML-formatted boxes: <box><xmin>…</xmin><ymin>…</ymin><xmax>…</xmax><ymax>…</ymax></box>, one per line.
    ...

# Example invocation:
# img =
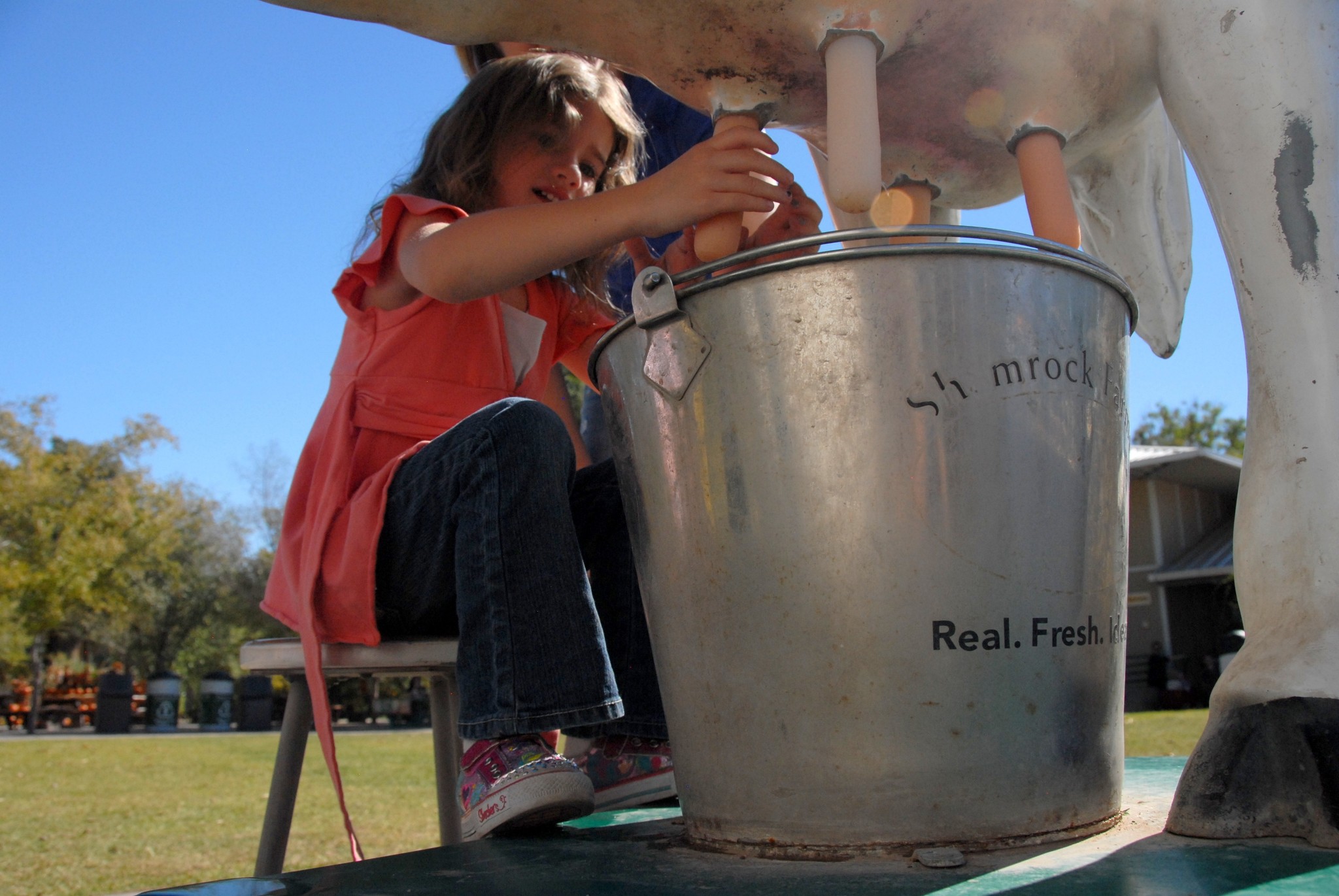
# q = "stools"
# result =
<box><xmin>235</xmin><ymin>637</ymin><xmax>464</xmax><ymax>876</ymax></box>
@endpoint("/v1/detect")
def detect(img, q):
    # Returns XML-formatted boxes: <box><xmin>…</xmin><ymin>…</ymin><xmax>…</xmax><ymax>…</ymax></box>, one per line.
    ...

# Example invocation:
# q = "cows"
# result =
<box><xmin>261</xmin><ymin>0</ymin><xmax>1338</xmax><ymax>850</ymax></box>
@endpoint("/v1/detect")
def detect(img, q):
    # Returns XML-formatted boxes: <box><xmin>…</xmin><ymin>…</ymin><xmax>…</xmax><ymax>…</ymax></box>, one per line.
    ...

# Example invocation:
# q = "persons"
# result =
<box><xmin>260</xmin><ymin>53</ymin><xmax>823</xmax><ymax>862</ymax></box>
<box><xmin>457</xmin><ymin>41</ymin><xmax>713</xmax><ymax>462</ymax></box>
<box><xmin>1148</xmin><ymin>642</ymin><xmax>1219</xmax><ymax>710</ymax></box>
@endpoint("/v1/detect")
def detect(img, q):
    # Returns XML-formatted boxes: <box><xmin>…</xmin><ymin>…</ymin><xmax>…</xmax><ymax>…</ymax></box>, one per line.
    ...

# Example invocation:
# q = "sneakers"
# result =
<box><xmin>454</xmin><ymin>730</ymin><xmax>596</xmax><ymax>843</ymax></box>
<box><xmin>569</xmin><ymin>735</ymin><xmax>682</xmax><ymax>816</ymax></box>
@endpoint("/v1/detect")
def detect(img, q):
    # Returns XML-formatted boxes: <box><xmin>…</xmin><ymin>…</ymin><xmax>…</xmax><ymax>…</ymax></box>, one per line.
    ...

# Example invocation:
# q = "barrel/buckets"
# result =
<box><xmin>586</xmin><ymin>223</ymin><xmax>1141</xmax><ymax>859</ymax></box>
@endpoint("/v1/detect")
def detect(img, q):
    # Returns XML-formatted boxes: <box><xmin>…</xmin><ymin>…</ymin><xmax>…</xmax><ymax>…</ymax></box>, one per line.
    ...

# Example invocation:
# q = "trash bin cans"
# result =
<box><xmin>241</xmin><ymin>676</ymin><xmax>271</xmax><ymax>730</ymax></box>
<box><xmin>144</xmin><ymin>669</ymin><xmax>182</xmax><ymax>734</ymax></box>
<box><xmin>199</xmin><ymin>670</ymin><xmax>232</xmax><ymax>733</ymax></box>
<box><xmin>96</xmin><ymin>670</ymin><xmax>134</xmax><ymax>734</ymax></box>
